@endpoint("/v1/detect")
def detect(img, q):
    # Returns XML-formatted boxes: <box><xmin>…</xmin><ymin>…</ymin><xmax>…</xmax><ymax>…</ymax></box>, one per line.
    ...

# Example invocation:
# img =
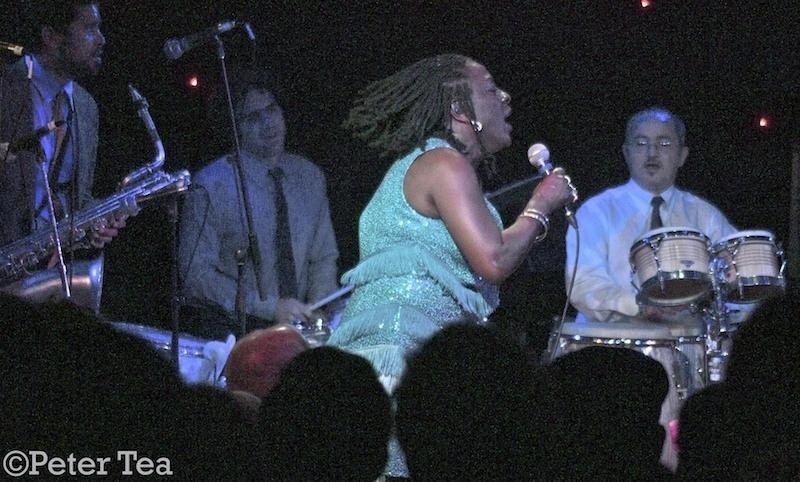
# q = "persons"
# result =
<box><xmin>565</xmin><ymin>105</ymin><xmax>740</xmax><ymax>322</ymax></box>
<box><xmin>323</xmin><ymin>54</ymin><xmax>578</xmax><ymax>481</ymax></box>
<box><xmin>0</xmin><ymin>0</ymin><xmax>126</xmax><ymax>268</ymax></box>
<box><xmin>174</xmin><ymin>80</ymin><xmax>340</xmax><ymax>324</ymax></box>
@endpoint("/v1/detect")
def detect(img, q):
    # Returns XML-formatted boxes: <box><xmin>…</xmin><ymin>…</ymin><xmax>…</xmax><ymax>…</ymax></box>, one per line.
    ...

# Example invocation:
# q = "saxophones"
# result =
<box><xmin>1</xmin><ymin>83</ymin><xmax>192</xmax><ymax>313</ymax></box>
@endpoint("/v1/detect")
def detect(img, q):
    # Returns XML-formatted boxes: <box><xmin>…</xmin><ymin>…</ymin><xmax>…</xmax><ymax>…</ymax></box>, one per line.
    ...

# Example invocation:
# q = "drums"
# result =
<box><xmin>711</xmin><ymin>230</ymin><xmax>785</xmax><ymax>305</ymax></box>
<box><xmin>106</xmin><ymin>321</ymin><xmax>218</xmax><ymax>386</ymax></box>
<box><xmin>629</xmin><ymin>226</ymin><xmax>715</xmax><ymax>307</ymax></box>
<box><xmin>549</xmin><ymin>318</ymin><xmax>710</xmax><ymax>473</ymax></box>
<box><xmin>712</xmin><ymin>311</ymin><xmax>756</xmax><ymax>381</ymax></box>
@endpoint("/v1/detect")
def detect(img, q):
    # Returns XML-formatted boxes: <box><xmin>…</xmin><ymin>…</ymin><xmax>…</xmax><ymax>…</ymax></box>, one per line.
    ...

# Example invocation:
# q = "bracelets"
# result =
<box><xmin>518</xmin><ymin>207</ymin><xmax>550</xmax><ymax>244</ymax></box>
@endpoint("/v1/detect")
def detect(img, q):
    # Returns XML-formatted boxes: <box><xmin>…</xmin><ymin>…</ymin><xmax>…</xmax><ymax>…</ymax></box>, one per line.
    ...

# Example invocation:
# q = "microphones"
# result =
<box><xmin>119</xmin><ymin>182</ymin><xmax>199</xmax><ymax>217</ymax></box>
<box><xmin>0</xmin><ymin>40</ymin><xmax>25</xmax><ymax>58</ymax></box>
<box><xmin>162</xmin><ymin>19</ymin><xmax>235</xmax><ymax>62</ymax></box>
<box><xmin>0</xmin><ymin>119</ymin><xmax>65</xmax><ymax>163</ymax></box>
<box><xmin>527</xmin><ymin>143</ymin><xmax>578</xmax><ymax>230</ymax></box>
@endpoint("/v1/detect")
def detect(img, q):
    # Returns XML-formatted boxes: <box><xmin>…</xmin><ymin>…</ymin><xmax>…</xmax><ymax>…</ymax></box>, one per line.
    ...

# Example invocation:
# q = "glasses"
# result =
<box><xmin>626</xmin><ymin>138</ymin><xmax>682</xmax><ymax>151</ymax></box>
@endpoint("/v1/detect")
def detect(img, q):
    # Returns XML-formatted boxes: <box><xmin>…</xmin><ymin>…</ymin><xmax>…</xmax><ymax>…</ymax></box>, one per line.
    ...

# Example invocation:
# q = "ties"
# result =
<box><xmin>270</xmin><ymin>168</ymin><xmax>299</xmax><ymax>300</ymax></box>
<box><xmin>45</xmin><ymin>89</ymin><xmax>69</xmax><ymax>183</ymax></box>
<box><xmin>647</xmin><ymin>194</ymin><xmax>667</xmax><ymax>230</ymax></box>
